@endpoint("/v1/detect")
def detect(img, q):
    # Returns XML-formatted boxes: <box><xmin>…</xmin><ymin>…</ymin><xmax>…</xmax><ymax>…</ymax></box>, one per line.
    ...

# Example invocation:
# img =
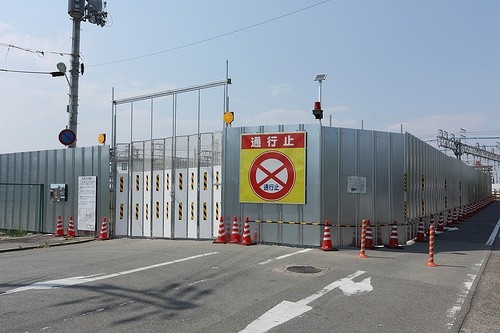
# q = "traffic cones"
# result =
<box><xmin>240</xmin><ymin>216</ymin><xmax>257</xmax><ymax>245</ymax></box>
<box><xmin>384</xmin><ymin>220</ymin><xmax>404</xmax><ymax>249</ymax></box>
<box><xmin>98</xmin><ymin>216</ymin><xmax>109</xmax><ymax>240</ymax></box>
<box><xmin>444</xmin><ymin>209</ymin><xmax>456</xmax><ymax>226</ymax></box>
<box><xmin>63</xmin><ymin>215</ymin><xmax>79</xmax><ymax>237</ymax></box>
<box><xmin>458</xmin><ymin>206</ymin><xmax>464</xmax><ymax>221</ymax></box>
<box><xmin>55</xmin><ymin>215</ymin><xmax>67</xmax><ymax>237</ymax></box>
<box><xmin>320</xmin><ymin>219</ymin><xmax>339</xmax><ymax>251</ymax></box>
<box><xmin>212</xmin><ymin>216</ymin><xmax>228</xmax><ymax>243</ymax></box>
<box><xmin>358</xmin><ymin>220</ymin><xmax>377</xmax><ymax>250</ymax></box>
<box><xmin>228</xmin><ymin>215</ymin><xmax>242</xmax><ymax>243</ymax></box>
<box><xmin>426</xmin><ymin>214</ymin><xmax>439</xmax><ymax>236</ymax></box>
<box><xmin>452</xmin><ymin>208</ymin><xmax>459</xmax><ymax>224</ymax></box>
<box><xmin>414</xmin><ymin>217</ymin><xmax>428</xmax><ymax>242</ymax></box>
<box><xmin>435</xmin><ymin>212</ymin><xmax>449</xmax><ymax>231</ymax></box>
<box><xmin>467</xmin><ymin>196</ymin><xmax>495</xmax><ymax>218</ymax></box>
<box><xmin>462</xmin><ymin>204</ymin><xmax>468</xmax><ymax>219</ymax></box>
